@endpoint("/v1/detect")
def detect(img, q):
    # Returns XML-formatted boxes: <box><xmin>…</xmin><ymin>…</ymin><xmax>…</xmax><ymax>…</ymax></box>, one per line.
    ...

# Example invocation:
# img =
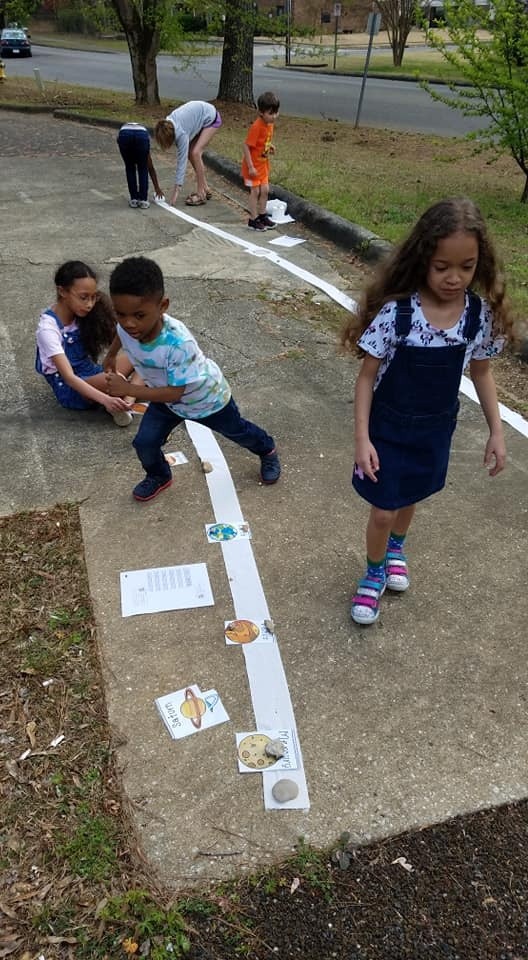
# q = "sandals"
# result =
<box><xmin>188</xmin><ymin>190</ymin><xmax>212</xmax><ymax>200</ymax></box>
<box><xmin>185</xmin><ymin>192</ymin><xmax>207</xmax><ymax>205</ymax></box>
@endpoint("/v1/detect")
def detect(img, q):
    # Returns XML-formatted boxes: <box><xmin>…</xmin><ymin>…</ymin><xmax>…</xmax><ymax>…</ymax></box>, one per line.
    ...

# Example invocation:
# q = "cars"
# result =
<box><xmin>0</xmin><ymin>27</ymin><xmax>32</xmax><ymax>58</ymax></box>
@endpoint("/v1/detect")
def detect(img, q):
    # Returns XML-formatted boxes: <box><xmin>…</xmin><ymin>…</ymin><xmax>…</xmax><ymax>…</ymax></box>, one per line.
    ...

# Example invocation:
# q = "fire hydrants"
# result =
<box><xmin>0</xmin><ymin>59</ymin><xmax>8</xmax><ymax>84</ymax></box>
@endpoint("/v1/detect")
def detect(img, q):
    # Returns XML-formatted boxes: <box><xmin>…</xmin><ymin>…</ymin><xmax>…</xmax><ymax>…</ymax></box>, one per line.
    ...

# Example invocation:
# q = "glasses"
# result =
<box><xmin>66</xmin><ymin>287</ymin><xmax>99</xmax><ymax>305</ymax></box>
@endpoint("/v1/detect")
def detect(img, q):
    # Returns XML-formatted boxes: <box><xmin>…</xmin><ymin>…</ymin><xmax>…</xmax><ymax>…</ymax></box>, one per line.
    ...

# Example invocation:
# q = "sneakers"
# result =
<box><xmin>352</xmin><ymin>577</ymin><xmax>387</xmax><ymax>622</ymax></box>
<box><xmin>385</xmin><ymin>551</ymin><xmax>409</xmax><ymax>592</ymax></box>
<box><xmin>259</xmin><ymin>213</ymin><xmax>277</xmax><ymax>228</ymax></box>
<box><xmin>248</xmin><ymin>217</ymin><xmax>267</xmax><ymax>231</ymax></box>
<box><xmin>131</xmin><ymin>477</ymin><xmax>171</xmax><ymax>501</ymax></box>
<box><xmin>260</xmin><ymin>445</ymin><xmax>280</xmax><ymax>483</ymax></box>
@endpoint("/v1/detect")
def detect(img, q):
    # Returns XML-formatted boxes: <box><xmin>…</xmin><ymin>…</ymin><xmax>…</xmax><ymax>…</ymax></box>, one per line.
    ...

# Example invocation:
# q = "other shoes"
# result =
<box><xmin>111</xmin><ymin>409</ymin><xmax>133</xmax><ymax>426</ymax></box>
<box><xmin>139</xmin><ymin>200</ymin><xmax>150</xmax><ymax>208</ymax></box>
<box><xmin>129</xmin><ymin>199</ymin><xmax>139</xmax><ymax>208</ymax></box>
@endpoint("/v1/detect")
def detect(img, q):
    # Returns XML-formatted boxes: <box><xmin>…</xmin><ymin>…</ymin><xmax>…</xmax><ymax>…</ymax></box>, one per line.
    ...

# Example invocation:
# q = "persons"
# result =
<box><xmin>102</xmin><ymin>257</ymin><xmax>281</xmax><ymax>501</ymax></box>
<box><xmin>36</xmin><ymin>260</ymin><xmax>146</xmax><ymax>426</ymax></box>
<box><xmin>241</xmin><ymin>91</ymin><xmax>280</xmax><ymax>231</ymax></box>
<box><xmin>117</xmin><ymin>122</ymin><xmax>165</xmax><ymax>209</ymax></box>
<box><xmin>156</xmin><ymin>101</ymin><xmax>222</xmax><ymax>206</ymax></box>
<box><xmin>339</xmin><ymin>197</ymin><xmax>507</xmax><ymax>624</ymax></box>
